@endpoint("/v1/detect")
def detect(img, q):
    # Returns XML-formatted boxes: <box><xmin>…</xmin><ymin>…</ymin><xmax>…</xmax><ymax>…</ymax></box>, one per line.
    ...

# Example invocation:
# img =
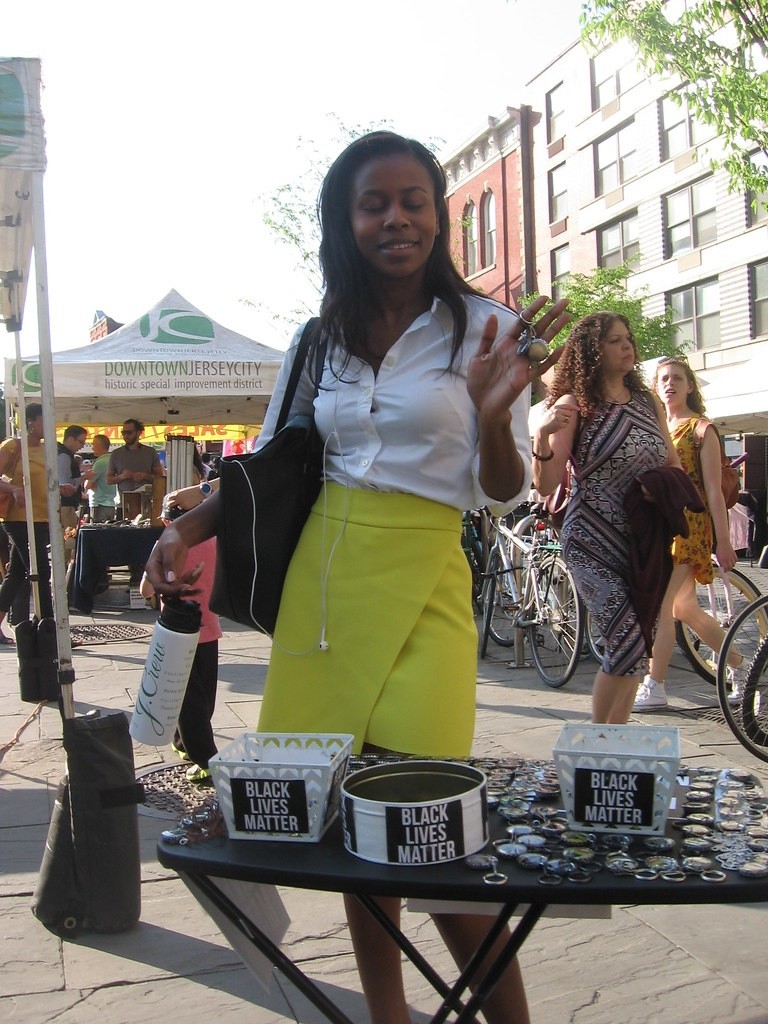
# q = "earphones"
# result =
<box><xmin>319</xmin><ymin>628</ymin><xmax>329</xmax><ymax>651</ymax></box>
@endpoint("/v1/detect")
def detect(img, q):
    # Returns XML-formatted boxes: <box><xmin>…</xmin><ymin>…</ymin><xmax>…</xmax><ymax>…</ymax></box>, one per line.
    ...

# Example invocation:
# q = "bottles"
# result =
<box><xmin>129</xmin><ymin>590</ymin><xmax>205</xmax><ymax>746</ymax></box>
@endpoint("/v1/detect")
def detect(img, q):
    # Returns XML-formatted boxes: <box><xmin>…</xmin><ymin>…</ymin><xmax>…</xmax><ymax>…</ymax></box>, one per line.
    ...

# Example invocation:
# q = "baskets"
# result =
<box><xmin>208</xmin><ymin>732</ymin><xmax>355</xmax><ymax>843</ymax></box>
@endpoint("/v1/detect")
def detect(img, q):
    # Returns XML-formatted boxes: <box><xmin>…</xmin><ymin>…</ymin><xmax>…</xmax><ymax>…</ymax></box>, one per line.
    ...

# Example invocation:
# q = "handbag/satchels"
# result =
<box><xmin>210</xmin><ymin>415</ymin><xmax>323</xmax><ymax>636</ymax></box>
<box><xmin>0</xmin><ymin>478</ymin><xmax>13</xmax><ymax>519</ymax></box>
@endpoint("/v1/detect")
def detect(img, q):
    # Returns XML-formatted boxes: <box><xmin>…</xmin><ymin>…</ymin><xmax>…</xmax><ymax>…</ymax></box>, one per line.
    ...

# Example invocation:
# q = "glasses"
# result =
<box><xmin>121</xmin><ymin>430</ymin><xmax>139</xmax><ymax>435</ymax></box>
<box><xmin>658</xmin><ymin>354</ymin><xmax>690</xmax><ymax>369</ymax></box>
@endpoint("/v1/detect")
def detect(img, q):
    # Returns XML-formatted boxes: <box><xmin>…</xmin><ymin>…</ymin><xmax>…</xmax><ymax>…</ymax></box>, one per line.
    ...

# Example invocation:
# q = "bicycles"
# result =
<box><xmin>462</xmin><ymin>451</ymin><xmax>768</xmax><ymax>762</ymax></box>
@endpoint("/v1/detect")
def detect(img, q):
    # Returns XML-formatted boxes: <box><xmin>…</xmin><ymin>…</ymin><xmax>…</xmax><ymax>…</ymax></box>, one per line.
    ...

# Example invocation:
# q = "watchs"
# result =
<box><xmin>198</xmin><ymin>480</ymin><xmax>213</xmax><ymax>498</ymax></box>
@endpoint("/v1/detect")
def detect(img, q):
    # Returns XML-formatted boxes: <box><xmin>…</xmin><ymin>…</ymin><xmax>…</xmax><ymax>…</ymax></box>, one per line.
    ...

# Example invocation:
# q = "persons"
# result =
<box><xmin>631</xmin><ymin>357</ymin><xmax>752</xmax><ymax>713</ymax></box>
<box><xmin>532</xmin><ymin>311</ymin><xmax>683</xmax><ymax>724</ymax></box>
<box><xmin>0</xmin><ymin>402</ymin><xmax>216</xmax><ymax>649</ymax></box>
<box><xmin>145</xmin><ymin>134</ymin><xmax>570</xmax><ymax>1024</ymax></box>
<box><xmin>139</xmin><ymin>476</ymin><xmax>222</xmax><ymax>779</ymax></box>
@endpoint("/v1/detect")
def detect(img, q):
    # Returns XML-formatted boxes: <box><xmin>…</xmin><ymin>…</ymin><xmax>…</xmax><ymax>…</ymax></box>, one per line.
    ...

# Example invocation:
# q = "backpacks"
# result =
<box><xmin>693</xmin><ymin>421</ymin><xmax>739</xmax><ymax>508</ymax></box>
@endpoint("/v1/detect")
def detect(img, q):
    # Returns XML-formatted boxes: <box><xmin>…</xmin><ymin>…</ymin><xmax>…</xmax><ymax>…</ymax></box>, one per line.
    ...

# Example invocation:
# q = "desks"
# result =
<box><xmin>67</xmin><ymin>519</ymin><xmax>168</xmax><ymax>614</ymax></box>
<box><xmin>155</xmin><ymin>752</ymin><xmax>768</xmax><ymax>1024</ymax></box>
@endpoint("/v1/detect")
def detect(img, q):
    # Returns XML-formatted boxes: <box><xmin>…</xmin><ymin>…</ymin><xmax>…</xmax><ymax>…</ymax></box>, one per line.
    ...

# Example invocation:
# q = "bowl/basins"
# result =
<box><xmin>340</xmin><ymin>760</ymin><xmax>492</xmax><ymax>866</ymax></box>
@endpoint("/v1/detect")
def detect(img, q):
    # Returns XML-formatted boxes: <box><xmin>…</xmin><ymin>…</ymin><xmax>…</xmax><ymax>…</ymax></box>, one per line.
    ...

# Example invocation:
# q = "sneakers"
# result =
<box><xmin>631</xmin><ymin>675</ymin><xmax>668</xmax><ymax>712</ymax></box>
<box><xmin>727</xmin><ymin>657</ymin><xmax>751</xmax><ymax>704</ymax></box>
<box><xmin>171</xmin><ymin>738</ymin><xmax>190</xmax><ymax>759</ymax></box>
<box><xmin>185</xmin><ymin>763</ymin><xmax>216</xmax><ymax>780</ymax></box>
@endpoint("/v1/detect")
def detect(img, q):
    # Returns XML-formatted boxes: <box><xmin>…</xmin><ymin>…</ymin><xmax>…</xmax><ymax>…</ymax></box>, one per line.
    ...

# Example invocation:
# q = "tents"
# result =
<box><xmin>5</xmin><ymin>288</ymin><xmax>286</xmax><ymax>440</ymax></box>
<box><xmin>0</xmin><ymin>56</ymin><xmax>74</xmax><ymax>724</ymax></box>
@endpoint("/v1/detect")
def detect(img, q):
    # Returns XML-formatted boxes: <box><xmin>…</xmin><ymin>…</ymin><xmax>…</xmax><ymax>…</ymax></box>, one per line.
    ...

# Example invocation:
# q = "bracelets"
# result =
<box><xmin>532</xmin><ymin>449</ymin><xmax>555</xmax><ymax>462</ymax></box>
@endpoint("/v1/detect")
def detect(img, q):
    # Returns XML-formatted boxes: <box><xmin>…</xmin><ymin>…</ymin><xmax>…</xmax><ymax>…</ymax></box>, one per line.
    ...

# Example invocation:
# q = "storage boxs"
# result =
<box><xmin>208</xmin><ymin>732</ymin><xmax>356</xmax><ymax>842</ymax></box>
<box><xmin>130</xmin><ymin>586</ymin><xmax>152</xmax><ymax>609</ymax></box>
<box><xmin>554</xmin><ymin>725</ymin><xmax>682</xmax><ymax>836</ymax></box>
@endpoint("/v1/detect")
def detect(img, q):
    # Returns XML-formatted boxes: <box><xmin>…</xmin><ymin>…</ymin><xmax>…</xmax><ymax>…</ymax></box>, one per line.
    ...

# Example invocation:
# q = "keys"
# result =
<box><xmin>516</xmin><ymin>310</ymin><xmax>548</xmax><ymax>371</ymax></box>
